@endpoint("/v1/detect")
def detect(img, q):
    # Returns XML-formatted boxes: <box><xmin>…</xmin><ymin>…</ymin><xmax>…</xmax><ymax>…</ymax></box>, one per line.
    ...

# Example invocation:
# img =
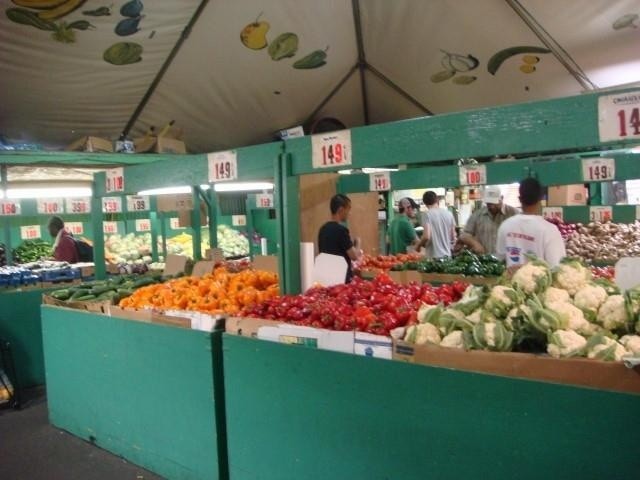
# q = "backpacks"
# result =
<box><xmin>61</xmin><ymin>237</ymin><xmax>93</xmax><ymax>262</ymax></box>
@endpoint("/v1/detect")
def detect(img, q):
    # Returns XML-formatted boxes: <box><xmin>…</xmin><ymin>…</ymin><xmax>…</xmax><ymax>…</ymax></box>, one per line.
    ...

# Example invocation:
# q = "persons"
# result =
<box><xmin>384</xmin><ymin>197</ymin><xmax>421</xmax><ymax>256</ymax></box>
<box><xmin>414</xmin><ymin>190</ymin><xmax>457</xmax><ymax>259</ymax></box>
<box><xmin>46</xmin><ymin>215</ymin><xmax>81</xmax><ymax>263</ymax></box>
<box><xmin>496</xmin><ymin>176</ymin><xmax>566</xmax><ymax>268</ymax></box>
<box><xmin>460</xmin><ymin>187</ymin><xmax>520</xmax><ymax>254</ymax></box>
<box><xmin>318</xmin><ymin>193</ymin><xmax>360</xmax><ymax>285</ymax></box>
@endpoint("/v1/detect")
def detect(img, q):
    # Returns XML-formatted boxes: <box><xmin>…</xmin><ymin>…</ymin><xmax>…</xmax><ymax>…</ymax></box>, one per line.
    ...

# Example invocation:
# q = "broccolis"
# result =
<box><xmin>403</xmin><ymin>251</ymin><xmax>640</xmax><ymax>361</ymax></box>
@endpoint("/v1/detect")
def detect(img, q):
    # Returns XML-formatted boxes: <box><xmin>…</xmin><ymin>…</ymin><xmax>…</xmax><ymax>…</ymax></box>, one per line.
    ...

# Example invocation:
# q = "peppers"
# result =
<box><xmin>392</xmin><ymin>250</ymin><xmax>506</xmax><ymax>276</ymax></box>
<box><xmin>12</xmin><ymin>239</ymin><xmax>55</xmax><ymax>264</ymax></box>
<box><xmin>239</xmin><ymin>271</ymin><xmax>468</xmax><ymax>339</ymax></box>
<box><xmin>119</xmin><ymin>267</ymin><xmax>279</xmax><ymax>317</ymax></box>
<box><xmin>350</xmin><ymin>250</ymin><xmax>419</xmax><ymax>271</ymax></box>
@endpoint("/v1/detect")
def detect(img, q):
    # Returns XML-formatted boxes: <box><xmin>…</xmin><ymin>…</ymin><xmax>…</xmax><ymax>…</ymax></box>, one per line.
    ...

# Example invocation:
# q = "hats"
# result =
<box><xmin>484</xmin><ymin>187</ymin><xmax>501</xmax><ymax>205</ymax></box>
<box><xmin>399</xmin><ymin>198</ymin><xmax>420</xmax><ymax>209</ymax></box>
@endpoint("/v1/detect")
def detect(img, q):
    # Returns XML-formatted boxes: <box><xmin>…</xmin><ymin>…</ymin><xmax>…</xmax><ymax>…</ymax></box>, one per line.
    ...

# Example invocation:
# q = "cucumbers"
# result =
<box><xmin>51</xmin><ymin>271</ymin><xmax>185</xmax><ymax>302</ymax></box>
